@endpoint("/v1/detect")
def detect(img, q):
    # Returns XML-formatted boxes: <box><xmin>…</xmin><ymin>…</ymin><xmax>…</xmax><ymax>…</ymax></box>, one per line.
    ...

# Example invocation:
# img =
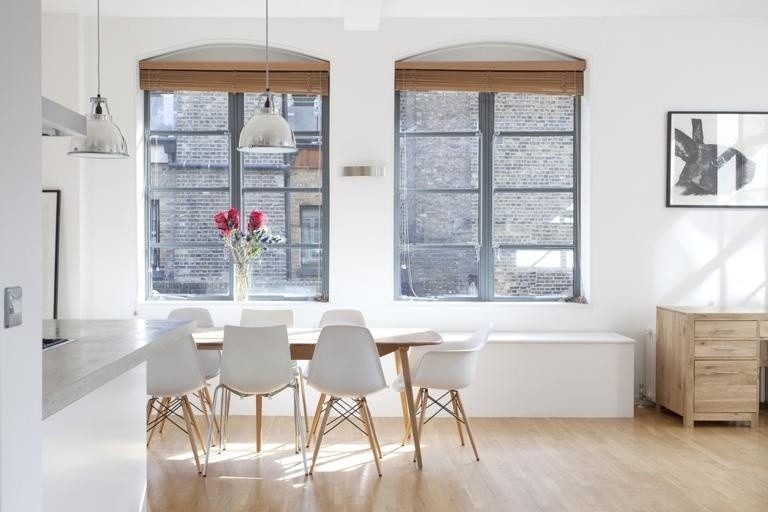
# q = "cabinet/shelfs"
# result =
<box><xmin>656</xmin><ymin>305</ymin><xmax>768</xmax><ymax>429</ymax></box>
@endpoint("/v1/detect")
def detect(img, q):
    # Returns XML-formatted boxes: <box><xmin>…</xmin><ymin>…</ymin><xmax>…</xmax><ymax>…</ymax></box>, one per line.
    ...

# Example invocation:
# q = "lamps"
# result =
<box><xmin>67</xmin><ymin>1</ymin><xmax>129</xmax><ymax>159</ymax></box>
<box><xmin>237</xmin><ymin>0</ymin><xmax>298</xmax><ymax>153</ymax></box>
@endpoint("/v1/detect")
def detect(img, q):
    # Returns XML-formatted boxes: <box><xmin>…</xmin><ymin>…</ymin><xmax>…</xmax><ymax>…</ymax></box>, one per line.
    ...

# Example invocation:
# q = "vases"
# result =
<box><xmin>233</xmin><ymin>263</ymin><xmax>249</xmax><ymax>303</ymax></box>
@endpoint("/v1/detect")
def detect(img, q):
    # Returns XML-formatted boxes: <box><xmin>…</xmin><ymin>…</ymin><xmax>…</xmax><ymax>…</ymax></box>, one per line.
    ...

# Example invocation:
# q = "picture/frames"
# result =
<box><xmin>666</xmin><ymin>110</ymin><xmax>767</xmax><ymax>209</ymax></box>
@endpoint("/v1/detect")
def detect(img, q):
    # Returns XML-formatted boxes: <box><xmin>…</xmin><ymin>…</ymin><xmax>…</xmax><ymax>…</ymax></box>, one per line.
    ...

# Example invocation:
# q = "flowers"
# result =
<box><xmin>213</xmin><ymin>207</ymin><xmax>283</xmax><ymax>263</ymax></box>
